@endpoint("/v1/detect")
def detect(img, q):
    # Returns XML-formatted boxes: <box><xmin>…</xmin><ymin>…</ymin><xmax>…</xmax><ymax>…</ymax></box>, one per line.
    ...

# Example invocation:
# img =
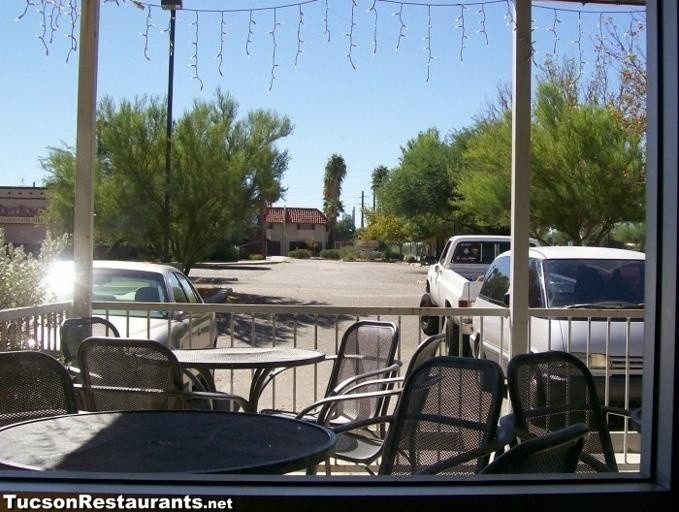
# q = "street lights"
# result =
<box><xmin>161</xmin><ymin>1</ymin><xmax>183</xmax><ymax>261</ymax></box>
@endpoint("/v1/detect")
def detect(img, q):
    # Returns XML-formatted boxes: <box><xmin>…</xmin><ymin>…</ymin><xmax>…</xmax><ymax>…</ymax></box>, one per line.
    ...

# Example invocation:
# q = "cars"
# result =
<box><xmin>25</xmin><ymin>260</ymin><xmax>217</xmax><ymax>391</ymax></box>
<box><xmin>469</xmin><ymin>245</ymin><xmax>645</xmax><ymax>414</ymax></box>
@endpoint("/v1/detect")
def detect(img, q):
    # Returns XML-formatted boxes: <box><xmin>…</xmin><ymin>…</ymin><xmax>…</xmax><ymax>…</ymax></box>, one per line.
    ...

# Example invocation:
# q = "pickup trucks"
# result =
<box><xmin>418</xmin><ymin>233</ymin><xmax>540</xmax><ymax>355</ymax></box>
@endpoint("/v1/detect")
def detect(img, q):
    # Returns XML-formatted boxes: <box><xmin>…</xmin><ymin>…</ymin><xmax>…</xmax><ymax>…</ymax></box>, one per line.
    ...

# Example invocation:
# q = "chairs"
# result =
<box><xmin>0</xmin><ymin>316</ymin><xmax>644</xmax><ymax>473</ymax></box>
